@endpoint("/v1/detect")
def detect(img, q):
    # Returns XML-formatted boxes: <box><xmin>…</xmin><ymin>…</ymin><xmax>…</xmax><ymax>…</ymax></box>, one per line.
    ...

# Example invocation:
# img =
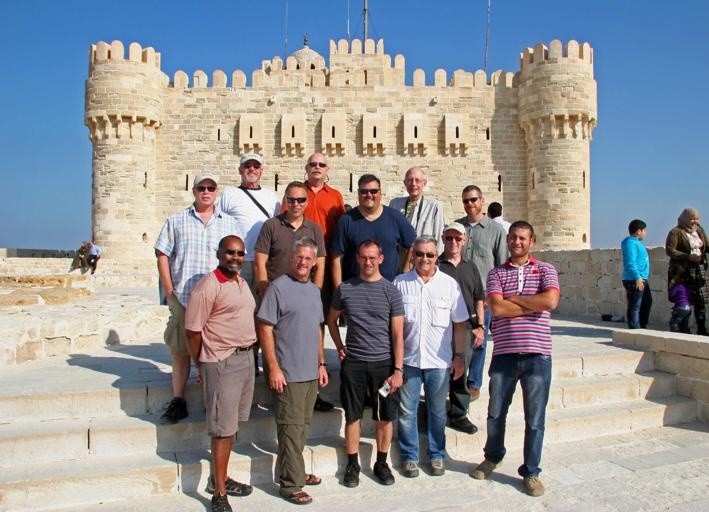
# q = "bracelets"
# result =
<box><xmin>318</xmin><ymin>363</ymin><xmax>329</xmax><ymax>367</ymax></box>
<box><xmin>456</xmin><ymin>352</ymin><xmax>465</xmax><ymax>359</ymax></box>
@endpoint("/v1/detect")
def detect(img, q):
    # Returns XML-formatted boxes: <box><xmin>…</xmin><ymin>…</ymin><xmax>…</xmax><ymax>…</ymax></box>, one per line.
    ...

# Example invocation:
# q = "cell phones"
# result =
<box><xmin>378</xmin><ymin>384</ymin><xmax>391</xmax><ymax>398</ymax></box>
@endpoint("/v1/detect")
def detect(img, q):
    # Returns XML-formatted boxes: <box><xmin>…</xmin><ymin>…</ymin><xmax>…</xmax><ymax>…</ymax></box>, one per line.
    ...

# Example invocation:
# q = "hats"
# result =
<box><xmin>194</xmin><ymin>172</ymin><xmax>218</xmax><ymax>189</ymax></box>
<box><xmin>240</xmin><ymin>153</ymin><xmax>263</xmax><ymax>165</ymax></box>
<box><xmin>443</xmin><ymin>222</ymin><xmax>466</xmax><ymax>236</ymax></box>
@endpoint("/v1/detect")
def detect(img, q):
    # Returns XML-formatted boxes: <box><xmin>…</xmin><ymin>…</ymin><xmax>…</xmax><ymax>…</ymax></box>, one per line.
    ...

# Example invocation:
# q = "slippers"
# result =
<box><xmin>287</xmin><ymin>491</ymin><xmax>312</xmax><ymax>504</ymax></box>
<box><xmin>305</xmin><ymin>475</ymin><xmax>321</xmax><ymax>485</ymax></box>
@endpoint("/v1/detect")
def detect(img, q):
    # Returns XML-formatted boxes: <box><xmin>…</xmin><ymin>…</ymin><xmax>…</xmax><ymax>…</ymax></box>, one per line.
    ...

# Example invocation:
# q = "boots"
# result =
<box><xmin>669</xmin><ymin>308</ymin><xmax>686</xmax><ymax>332</ymax></box>
<box><xmin>694</xmin><ymin>311</ymin><xmax>709</xmax><ymax>336</ymax></box>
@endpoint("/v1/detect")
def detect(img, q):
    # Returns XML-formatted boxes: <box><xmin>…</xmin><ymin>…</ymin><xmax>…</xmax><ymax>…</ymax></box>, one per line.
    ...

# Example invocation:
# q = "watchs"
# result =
<box><xmin>478</xmin><ymin>324</ymin><xmax>486</xmax><ymax>330</ymax></box>
<box><xmin>396</xmin><ymin>367</ymin><xmax>404</xmax><ymax>371</ymax></box>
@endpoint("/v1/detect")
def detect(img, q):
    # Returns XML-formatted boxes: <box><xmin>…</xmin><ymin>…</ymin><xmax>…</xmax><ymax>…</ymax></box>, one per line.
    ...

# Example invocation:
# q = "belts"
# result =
<box><xmin>235</xmin><ymin>344</ymin><xmax>253</xmax><ymax>352</ymax></box>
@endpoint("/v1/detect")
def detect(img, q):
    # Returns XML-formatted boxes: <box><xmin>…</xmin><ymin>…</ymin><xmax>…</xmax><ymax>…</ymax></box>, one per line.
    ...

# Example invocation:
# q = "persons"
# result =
<box><xmin>453</xmin><ymin>186</ymin><xmax>513</xmax><ymax>401</ymax></box>
<box><xmin>279</xmin><ymin>154</ymin><xmax>352</xmax><ymax>237</ymax></box>
<box><xmin>621</xmin><ymin>219</ymin><xmax>652</xmax><ymax>329</ymax></box>
<box><xmin>665</xmin><ymin>208</ymin><xmax>709</xmax><ymax>336</ymax></box>
<box><xmin>86</xmin><ymin>242</ymin><xmax>101</xmax><ymax>274</ymax></box>
<box><xmin>155</xmin><ymin>171</ymin><xmax>247</xmax><ymax>425</ymax></box>
<box><xmin>468</xmin><ymin>220</ymin><xmax>559</xmax><ymax>497</ymax></box>
<box><xmin>327</xmin><ymin>240</ymin><xmax>405</xmax><ymax>488</ymax></box>
<box><xmin>668</xmin><ymin>272</ymin><xmax>692</xmax><ymax>333</ymax></box>
<box><xmin>388</xmin><ymin>166</ymin><xmax>450</xmax><ymax>259</ymax></box>
<box><xmin>436</xmin><ymin>222</ymin><xmax>486</xmax><ymax>436</ymax></box>
<box><xmin>329</xmin><ymin>175</ymin><xmax>416</xmax><ymax>287</ymax></box>
<box><xmin>257</xmin><ymin>238</ymin><xmax>330</xmax><ymax>505</ymax></box>
<box><xmin>78</xmin><ymin>242</ymin><xmax>87</xmax><ymax>267</ymax></box>
<box><xmin>390</xmin><ymin>236</ymin><xmax>470</xmax><ymax>478</ymax></box>
<box><xmin>216</xmin><ymin>153</ymin><xmax>280</xmax><ymax>308</ymax></box>
<box><xmin>185</xmin><ymin>235</ymin><xmax>257</xmax><ymax>511</ymax></box>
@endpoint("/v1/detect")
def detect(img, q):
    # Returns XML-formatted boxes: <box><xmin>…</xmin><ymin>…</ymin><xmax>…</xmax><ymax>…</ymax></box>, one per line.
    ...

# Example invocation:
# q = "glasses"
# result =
<box><xmin>309</xmin><ymin>162</ymin><xmax>326</xmax><ymax>167</ymax></box>
<box><xmin>224</xmin><ymin>249</ymin><xmax>245</xmax><ymax>256</ymax></box>
<box><xmin>358</xmin><ymin>189</ymin><xmax>378</xmax><ymax>194</ymax></box>
<box><xmin>445</xmin><ymin>236</ymin><xmax>462</xmax><ymax>241</ymax></box>
<box><xmin>463</xmin><ymin>197</ymin><xmax>480</xmax><ymax>204</ymax></box>
<box><xmin>360</xmin><ymin>255</ymin><xmax>378</xmax><ymax>261</ymax></box>
<box><xmin>416</xmin><ymin>251</ymin><xmax>434</xmax><ymax>258</ymax></box>
<box><xmin>285</xmin><ymin>195</ymin><xmax>306</xmax><ymax>203</ymax></box>
<box><xmin>196</xmin><ymin>186</ymin><xmax>215</xmax><ymax>192</ymax></box>
<box><xmin>244</xmin><ymin>162</ymin><xmax>260</xmax><ymax>169</ymax></box>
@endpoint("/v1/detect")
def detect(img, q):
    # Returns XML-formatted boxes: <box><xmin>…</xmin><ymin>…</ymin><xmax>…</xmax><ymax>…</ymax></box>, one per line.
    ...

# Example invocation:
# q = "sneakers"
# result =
<box><xmin>468</xmin><ymin>386</ymin><xmax>479</xmax><ymax>401</ymax></box>
<box><xmin>314</xmin><ymin>397</ymin><xmax>334</xmax><ymax>411</ymax></box>
<box><xmin>344</xmin><ymin>462</ymin><xmax>360</xmax><ymax>487</ymax></box>
<box><xmin>470</xmin><ymin>458</ymin><xmax>503</xmax><ymax>480</ymax></box>
<box><xmin>374</xmin><ymin>462</ymin><xmax>394</xmax><ymax>485</ymax></box>
<box><xmin>523</xmin><ymin>476</ymin><xmax>545</xmax><ymax>497</ymax></box>
<box><xmin>429</xmin><ymin>459</ymin><xmax>445</xmax><ymax>476</ymax></box>
<box><xmin>399</xmin><ymin>462</ymin><xmax>419</xmax><ymax>477</ymax></box>
<box><xmin>159</xmin><ymin>397</ymin><xmax>189</xmax><ymax>424</ymax></box>
<box><xmin>449</xmin><ymin>417</ymin><xmax>477</xmax><ymax>434</ymax></box>
<box><xmin>207</xmin><ymin>475</ymin><xmax>253</xmax><ymax>512</ymax></box>
